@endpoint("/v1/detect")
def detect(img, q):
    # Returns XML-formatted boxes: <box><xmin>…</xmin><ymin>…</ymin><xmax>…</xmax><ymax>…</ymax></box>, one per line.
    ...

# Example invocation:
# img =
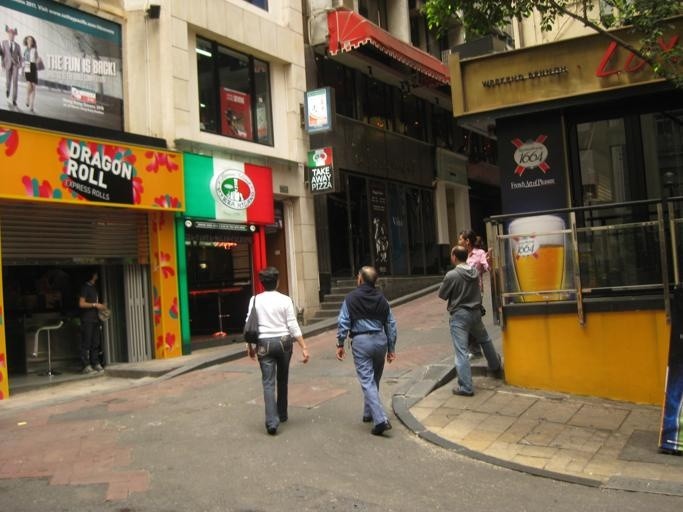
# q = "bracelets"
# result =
<box><xmin>93</xmin><ymin>304</ymin><xmax>96</xmax><ymax>307</ymax></box>
<box><xmin>337</xmin><ymin>345</ymin><xmax>343</xmax><ymax>349</ymax></box>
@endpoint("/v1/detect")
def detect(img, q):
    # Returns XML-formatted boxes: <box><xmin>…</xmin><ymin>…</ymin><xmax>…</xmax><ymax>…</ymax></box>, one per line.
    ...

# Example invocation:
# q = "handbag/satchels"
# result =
<box><xmin>242</xmin><ymin>308</ymin><xmax>258</xmax><ymax>343</ymax></box>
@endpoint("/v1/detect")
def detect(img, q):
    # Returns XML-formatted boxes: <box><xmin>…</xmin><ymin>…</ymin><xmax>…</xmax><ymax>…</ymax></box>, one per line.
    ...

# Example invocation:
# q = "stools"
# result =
<box><xmin>208</xmin><ymin>292</ymin><xmax>233</xmax><ymax>346</ymax></box>
<box><xmin>33</xmin><ymin>321</ymin><xmax>64</xmax><ymax>377</ymax></box>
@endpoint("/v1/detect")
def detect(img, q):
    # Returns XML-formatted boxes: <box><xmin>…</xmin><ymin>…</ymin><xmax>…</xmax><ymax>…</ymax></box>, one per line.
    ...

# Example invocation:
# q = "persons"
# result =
<box><xmin>22</xmin><ymin>35</ymin><xmax>40</xmax><ymax>112</ymax></box>
<box><xmin>1</xmin><ymin>25</ymin><xmax>21</xmax><ymax>106</ymax></box>
<box><xmin>245</xmin><ymin>267</ymin><xmax>310</xmax><ymax>434</ymax></box>
<box><xmin>78</xmin><ymin>268</ymin><xmax>108</xmax><ymax>375</ymax></box>
<box><xmin>336</xmin><ymin>266</ymin><xmax>397</xmax><ymax>435</ymax></box>
<box><xmin>459</xmin><ymin>229</ymin><xmax>492</xmax><ymax>360</ymax></box>
<box><xmin>438</xmin><ymin>244</ymin><xmax>500</xmax><ymax>397</ymax></box>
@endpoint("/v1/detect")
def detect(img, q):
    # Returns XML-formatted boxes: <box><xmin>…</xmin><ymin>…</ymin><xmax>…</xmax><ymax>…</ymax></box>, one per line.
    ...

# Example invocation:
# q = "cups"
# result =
<box><xmin>508</xmin><ymin>215</ymin><xmax>567</xmax><ymax>301</ymax></box>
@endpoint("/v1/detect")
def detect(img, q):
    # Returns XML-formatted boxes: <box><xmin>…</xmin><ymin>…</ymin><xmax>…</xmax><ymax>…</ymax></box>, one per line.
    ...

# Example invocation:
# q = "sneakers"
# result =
<box><xmin>84</xmin><ymin>364</ymin><xmax>103</xmax><ymax>375</ymax></box>
<box><xmin>453</xmin><ymin>387</ymin><xmax>472</xmax><ymax>395</ymax></box>
<box><xmin>363</xmin><ymin>416</ymin><xmax>373</xmax><ymax>423</ymax></box>
<box><xmin>268</xmin><ymin>427</ymin><xmax>277</xmax><ymax>436</ymax></box>
<box><xmin>468</xmin><ymin>353</ymin><xmax>482</xmax><ymax>360</ymax></box>
<box><xmin>371</xmin><ymin>421</ymin><xmax>392</xmax><ymax>435</ymax></box>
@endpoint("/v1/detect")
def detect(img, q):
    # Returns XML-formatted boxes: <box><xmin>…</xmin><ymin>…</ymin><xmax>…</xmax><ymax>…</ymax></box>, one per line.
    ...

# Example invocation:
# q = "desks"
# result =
<box><xmin>189</xmin><ymin>288</ymin><xmax>242</xmax><ymax>336</ymax></box>
